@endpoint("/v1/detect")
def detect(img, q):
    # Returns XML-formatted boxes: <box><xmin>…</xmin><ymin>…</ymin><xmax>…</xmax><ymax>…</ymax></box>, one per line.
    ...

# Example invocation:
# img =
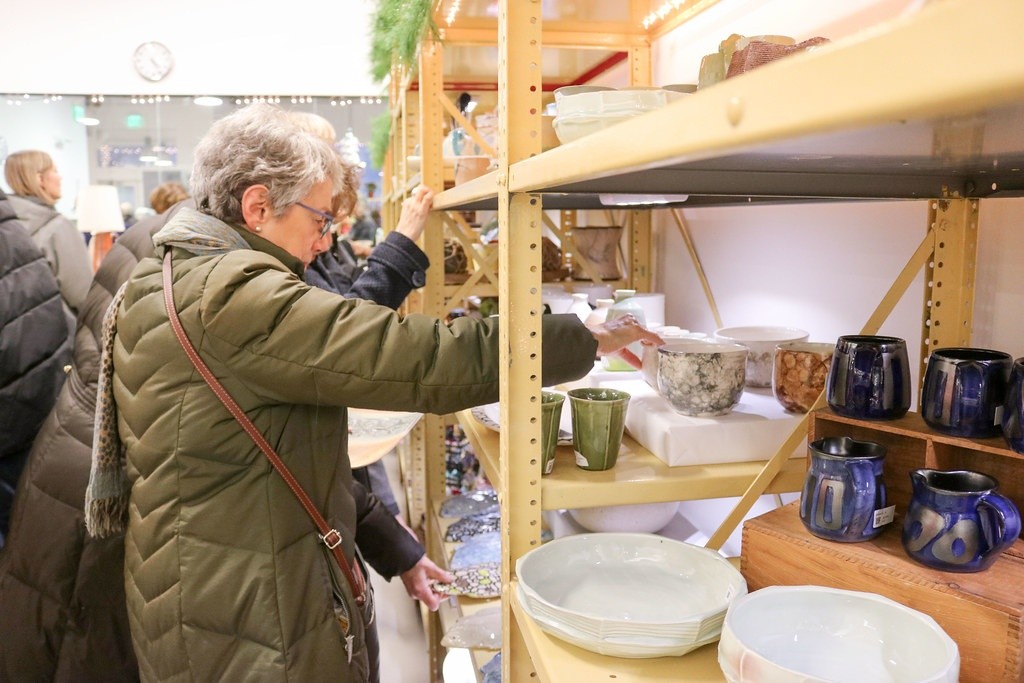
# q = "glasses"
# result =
<box><xmin>295</xmin><ymin>200</ymin><xmax>336</xmax><ymax>239</ymax></box>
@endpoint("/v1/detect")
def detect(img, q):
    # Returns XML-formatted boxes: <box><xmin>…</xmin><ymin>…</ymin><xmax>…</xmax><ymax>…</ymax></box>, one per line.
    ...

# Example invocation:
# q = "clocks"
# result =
<box><xmin>134</xmin><ymin>41</ymin><xmax>173</xmax><ymax>82</ymax></box>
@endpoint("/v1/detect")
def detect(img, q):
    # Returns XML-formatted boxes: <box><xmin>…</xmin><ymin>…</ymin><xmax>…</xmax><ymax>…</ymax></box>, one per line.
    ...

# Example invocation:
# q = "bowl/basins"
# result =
<box><xmin>554</xmin><ymin>85</ymin><xmax>698</xmax><ymax>144</ymax></box>
<box><xmin>454</xmin><ymin>156</ymin><xmax>491</xmax><ymax>186</ymax></box>
<box><xmin>347</xmin><ymin>407</ymin><xmax>423</xmax><ymax>468</ymax></box>
<box><xmin>714</xmin><ymin>326</ymin><xmax>809</xmax><ymax>388</ymax></box>
<box><xmin>718</xmin><ymin>585</ymin><xmax>960</xmax><ymax>683</ymax></box>
<box><xmin>657</xmin><ymin>342</ymin><xmax>749</xmax><ymax>417</ymax></box>
<box><xmin>699</xmin><ymin>53</ymin><xmax>724</xmax><ymax>90</ymax></box>
<box><xmin>772</xmin><ymin>342</ymin><xmax>836</xmax><ymax>413</ymax></box>
<box><xmin>515</xmin><ymin>532</ymin><xmax>748</xmax><ymax>658</ymax></box>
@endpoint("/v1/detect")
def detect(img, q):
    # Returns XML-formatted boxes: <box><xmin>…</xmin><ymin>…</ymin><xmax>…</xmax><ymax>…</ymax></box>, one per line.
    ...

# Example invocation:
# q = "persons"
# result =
<box><xmin>0</xmin><ymin>148</ymin><xmax>458</xmax><ymax>683</ymax></box>
<box><xmin>110</xmin><ymin>103</ymin><xmax>665</xmax><ymax>683</ymax></box>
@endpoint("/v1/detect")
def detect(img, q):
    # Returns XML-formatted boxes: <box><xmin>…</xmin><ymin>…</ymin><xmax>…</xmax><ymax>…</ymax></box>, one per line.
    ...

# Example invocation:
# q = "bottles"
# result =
<box><xmin>584</xmin><ymin>299</ymin><xmax>615</xmax><ymax>328</ymax></box>
<box><xmin>642</xmin><ymin>319</ymin><xmax>717</xmax><ymax>393</ymax></box>
<box><xmin>602</xmin><ymin>290</ymin><xmax>644</xmax><ymax>371</ymax></box>
<box><xmin>566</xmin><ymin>293</ymin><xmax>593</xmax><ymax>323</ymax></box>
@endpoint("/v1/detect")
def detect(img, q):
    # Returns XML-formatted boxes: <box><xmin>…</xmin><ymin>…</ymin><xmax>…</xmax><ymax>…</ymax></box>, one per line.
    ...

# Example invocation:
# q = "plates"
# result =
<box><xmin>471</xmin><ymin>385</ymin><xmax>574</xmax><ymax>445</ymax></box>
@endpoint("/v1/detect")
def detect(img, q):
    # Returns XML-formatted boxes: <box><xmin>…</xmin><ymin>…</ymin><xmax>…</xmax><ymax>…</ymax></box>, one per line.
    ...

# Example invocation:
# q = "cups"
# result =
<box><xmin>567</xmin><ymin>388</ymin><xmax>631</xmax><ymax>471</ymax></box>
<box><xmin>799</xmin><ymin>437</ymin><xmax>889</xmax><ymax>542</ymax></box>
<box><xmin>901</xmin><ymin>468</ymin><xmax>1021</xmax><ymax>572</ymax></box>
<box><xmin>826</xmin><ymin>335</ymin><xmax>911</xmax><ymax>420</ymax></box>
<box><xmin>1001</xmin><ymin>357</ymin><xmax>1024</xmax><ymax>455</ymax></box>
<box><xmin>922</xmin><ymin>347</ymin><xmax>1012</xmax><ymax>439</ymax></box>
<box><xmin>541</xmin><ymin>391</ymin><xmax>565</xmax><ymax>474</ymax></box>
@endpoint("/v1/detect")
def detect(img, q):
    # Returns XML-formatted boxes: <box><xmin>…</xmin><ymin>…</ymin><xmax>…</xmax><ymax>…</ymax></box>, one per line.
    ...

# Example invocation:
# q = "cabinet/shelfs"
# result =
<box><xmin>382</xmin><ymin>0</ymin><xmax>1024</xmax><ymax>683</ymax></box>
